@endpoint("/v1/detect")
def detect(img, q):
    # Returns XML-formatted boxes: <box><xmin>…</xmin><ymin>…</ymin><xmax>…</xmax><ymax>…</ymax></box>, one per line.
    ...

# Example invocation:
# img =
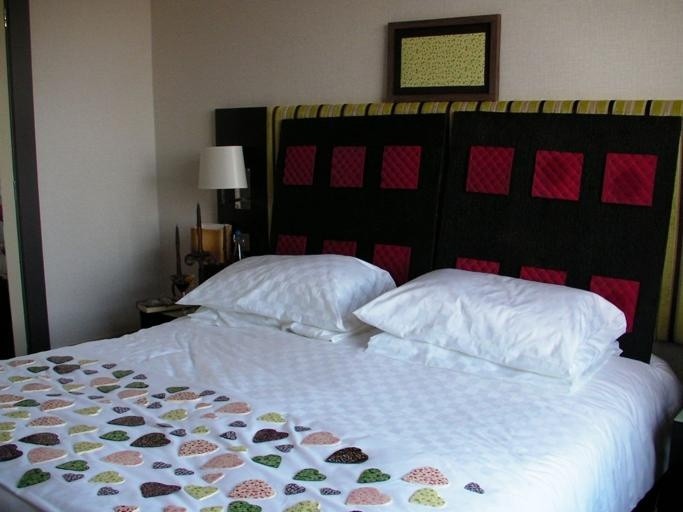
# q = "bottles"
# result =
<box><xmin>230</xmin><ymin>229</ymin><xmax>244</xmax><ymax>263</ymax></box>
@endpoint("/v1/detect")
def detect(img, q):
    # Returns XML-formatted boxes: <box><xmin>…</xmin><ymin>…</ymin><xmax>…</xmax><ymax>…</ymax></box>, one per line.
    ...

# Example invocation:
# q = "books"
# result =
<box><xmin>134</xmin><ymin>298</ymin><xmax>189</xmax><ymax>315</ymax></box>
<box><xmin>190</xmin><ymin>220</ymin><xmax>235</xmax><ymax>264</ymax></box>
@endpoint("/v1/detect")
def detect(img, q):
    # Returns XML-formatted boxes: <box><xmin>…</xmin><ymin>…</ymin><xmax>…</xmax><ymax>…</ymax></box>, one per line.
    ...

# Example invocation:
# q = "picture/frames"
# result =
<box><xmin>384</xmin><ymin>14</ymin><xmax>503</xmax><ymax>107</ymax></box>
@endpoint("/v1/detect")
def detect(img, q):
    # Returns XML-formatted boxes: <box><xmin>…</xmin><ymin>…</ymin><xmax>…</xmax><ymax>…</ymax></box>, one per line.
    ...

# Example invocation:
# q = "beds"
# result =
<box><xmin>2</xmin><ymin>103</ymin><xmax>683</xmax><ymax>511</ymax></box>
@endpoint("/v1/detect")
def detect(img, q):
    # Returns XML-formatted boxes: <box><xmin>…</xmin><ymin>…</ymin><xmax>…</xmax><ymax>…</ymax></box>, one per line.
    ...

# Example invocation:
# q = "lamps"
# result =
<box><xmin>195</xmin><ymin>143</ymin><xmax>255</xmax><ymax>217</ymax></box>
